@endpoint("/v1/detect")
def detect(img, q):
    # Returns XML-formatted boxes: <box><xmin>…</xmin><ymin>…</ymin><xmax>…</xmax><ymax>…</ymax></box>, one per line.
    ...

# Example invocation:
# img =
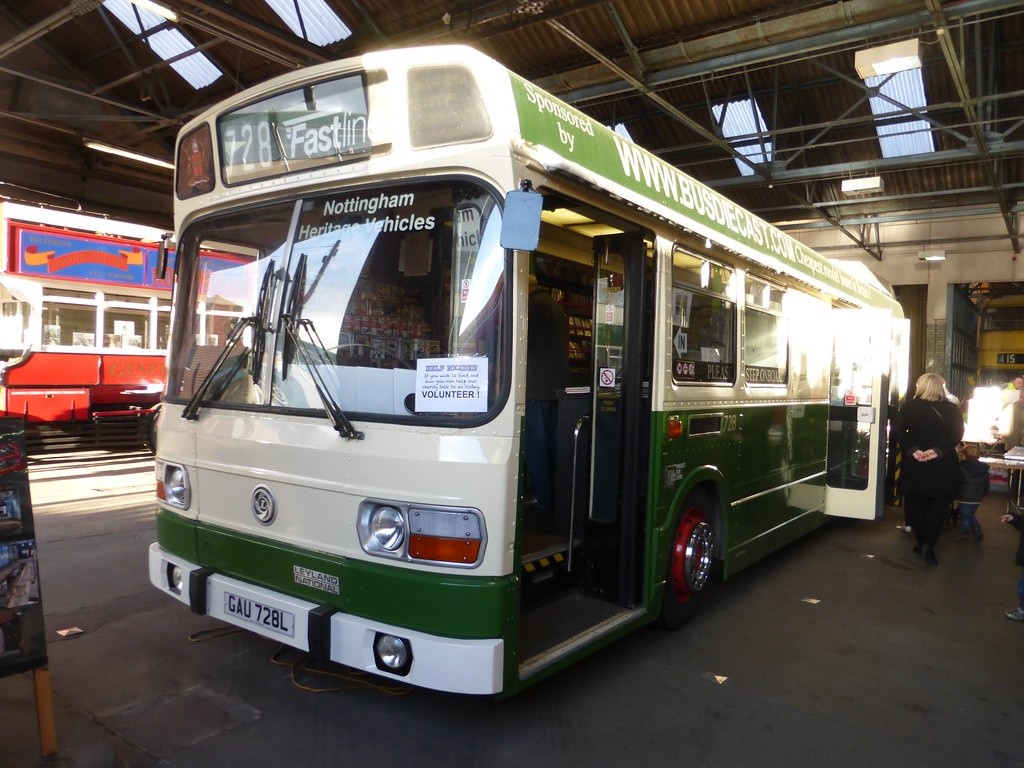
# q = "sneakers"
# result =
<box><xmin>1005</xmin><ymin>607</ymin><xmax>1024</xmax><ymax>620</ymax></box>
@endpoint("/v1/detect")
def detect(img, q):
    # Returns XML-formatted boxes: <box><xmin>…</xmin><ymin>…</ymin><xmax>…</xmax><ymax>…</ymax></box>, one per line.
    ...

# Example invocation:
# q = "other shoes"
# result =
<box><xmin>905</xmin><ymin>525</ymin><xmax>912</xmax><ymax>533</ymax></box>
<box><xmin>955</xmin><ymin>534</ymin><xmax>969</xmax><ymax>544</ymax></box>
<box><xmin>942</xmin><ymin>524</ymin><xmax>948</xmax><ymax>530</ymax></box>
<box><xmin>976</xmin><ymin>532</ymin><xmax>984</xmax><ymax>542</ymax></box>
<box><xmin>913</xmin><ymin>546</ymin><xmax>924</xmax><ymax>556</ymax></box>
<box><xmin>926</xmin><ymin>550</ymin><xmax>937</xmax><ymax>565</ymax></box>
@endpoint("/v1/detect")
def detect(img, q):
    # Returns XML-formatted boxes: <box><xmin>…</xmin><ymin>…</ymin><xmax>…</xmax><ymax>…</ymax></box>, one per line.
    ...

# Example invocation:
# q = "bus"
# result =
<box><xmin>1</xmin><ymin>183</ymin><xmax>264</xmax><ymax>456</ymax></box>
<box><xmin>148</xmin><ymin>46</ymin><xmax>912</xmax><ymax>701</ymax></box>
<box><xmin>975</xmin><ymin>295</ymin><xmax>1024</xmax><ymax>455</ymax></box>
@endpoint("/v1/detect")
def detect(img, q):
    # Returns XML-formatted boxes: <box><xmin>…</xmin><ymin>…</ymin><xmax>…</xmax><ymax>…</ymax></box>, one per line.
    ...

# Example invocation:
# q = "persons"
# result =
<box><xmin>893</xmin><ymin>372</ymin><xmax>1024</xmax><ymax>621</ymax></box>
<box><xmin>525</xmin><ymin>274</ymin><xmax>571</xmax><ymax>509</ymax></box>
